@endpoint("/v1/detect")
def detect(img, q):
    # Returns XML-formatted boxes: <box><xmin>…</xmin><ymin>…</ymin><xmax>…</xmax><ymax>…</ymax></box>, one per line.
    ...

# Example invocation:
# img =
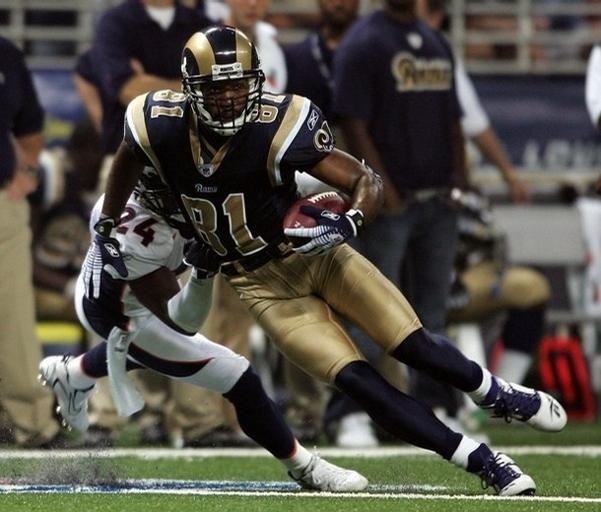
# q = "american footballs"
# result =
<box><xmin>283</xmin><ymin>192</ymin><xmax>350</xmax><ymax>248</ymax></box>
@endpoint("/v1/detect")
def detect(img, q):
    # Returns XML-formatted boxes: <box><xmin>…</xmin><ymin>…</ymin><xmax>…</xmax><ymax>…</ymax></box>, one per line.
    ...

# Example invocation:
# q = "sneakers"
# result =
<box><xmin>476</xmin><ymin>368</ymin><xmax>568</xmax><ymax>433</ymax></box>
<box><xmin>286</xmin><ymin>445</ymin><xmax>370</xmax><ymax>492</ymax></box>
<box><xmin>38</xmin><ymin>353</ymin><xmax>94</xmax><ymax>435</ymax></box>
<box><xmin>436</xmin><ymin>409</ymin><xmax>465</xmax><ymax>441</ymax></box>
<box><xmin>466</xmin><ymin>440</ymin><xmax>539</xmax><ymax>499</ymax></box>
<box><xmin>332</xmin><ymin>409</ymin><xmax>378</xmax><ymax>447</ymax></box>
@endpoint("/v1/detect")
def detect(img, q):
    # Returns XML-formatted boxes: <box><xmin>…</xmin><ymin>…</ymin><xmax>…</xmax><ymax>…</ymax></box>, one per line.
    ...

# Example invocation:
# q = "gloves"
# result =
<box><xmin>79</xmin><ymin>233</ymin><xmax>128</xmax><ymax>301</ymax></box>
<box><xmin>179</xmin><ymin>243</ymin><xmax>220</xmax><ymax>279</ymax></box>
<box><xmin>283</xmin><ymin>208</ymin><xmax>365</xmax><ymax>259</ymax></box>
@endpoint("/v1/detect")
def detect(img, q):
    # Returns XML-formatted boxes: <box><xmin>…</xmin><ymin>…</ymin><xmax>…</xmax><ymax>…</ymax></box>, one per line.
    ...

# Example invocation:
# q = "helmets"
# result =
<box><xmin>135</xmin><ymin>164</ymin><xmax>192</xmax><ymax>230</ymax></box>
<box><xmin>179</xmin><ymin>23</ymin><xmax>266</xmax><ymax>137</ymax></box>
